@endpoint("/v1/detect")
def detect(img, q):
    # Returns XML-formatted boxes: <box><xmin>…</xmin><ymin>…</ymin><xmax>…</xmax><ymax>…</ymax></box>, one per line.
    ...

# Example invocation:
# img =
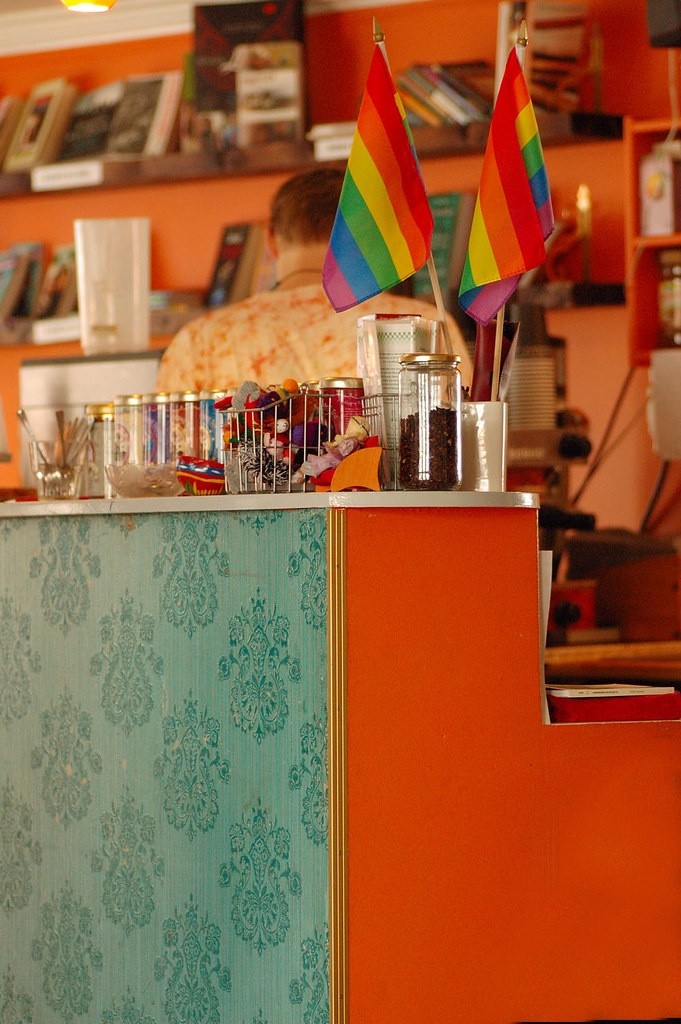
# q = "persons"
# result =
<box><xmin>154</xmin><ymin>168</ymin><xmax>473</xmax><ymax>401</ymax></box>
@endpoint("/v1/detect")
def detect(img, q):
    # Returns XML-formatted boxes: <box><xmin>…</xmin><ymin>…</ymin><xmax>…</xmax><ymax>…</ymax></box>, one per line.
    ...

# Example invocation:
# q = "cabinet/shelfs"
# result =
<box><xmin>0</xmin><ymin>116</ymin><xmax>625</xmax><ymax>377</ymax></box>
<box><xmin>626</xmin><ymin>114</ymin><xmax>681</xmax><ymax>538</ymax></box>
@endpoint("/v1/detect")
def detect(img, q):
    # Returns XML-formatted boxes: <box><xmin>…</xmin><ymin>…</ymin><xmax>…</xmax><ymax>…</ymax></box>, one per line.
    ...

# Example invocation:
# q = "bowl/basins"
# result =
<box><xmin>105</xmin><ymin>463</ymin><xmax>186</xmax><ymax>497</ymax></box>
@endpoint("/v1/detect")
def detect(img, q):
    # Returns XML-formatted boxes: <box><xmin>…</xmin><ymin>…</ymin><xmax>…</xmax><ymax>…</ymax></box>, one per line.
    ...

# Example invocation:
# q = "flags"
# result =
<box><xmin>455</xmin><ymin>45</ymin><xmax>554</xmax><ymax>329</ymax></box>
<box><xmin>321</xmin><ymin>45</ymin><xmax>434</xmax><ymax>315</ymax></box>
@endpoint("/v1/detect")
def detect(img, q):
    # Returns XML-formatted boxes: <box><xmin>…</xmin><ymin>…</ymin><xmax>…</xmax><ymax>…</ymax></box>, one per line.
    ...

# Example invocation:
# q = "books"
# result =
<box><xmin>1</xmin><ymin>240</ymin><xmax>80</xmax><ymax>319</ymax></box>
<box><xmin>546</xmin><ymin>683</ymin><xmax>675</xmax><ymax>698</ymax></box>
<box><xmin>181</xmin><ymin>41</ymin><xmax>302</xmax><ymax>169</ymax></box>
<box><xmin>396</xmin><ymin>60</ymin><xmax>495</xmax><ymax>130</ymax></box>
<box><xmin>0</xmin><ymin>69</ymin><xmax>214</xmax><ymax>189</ymax></box>
<box><xmin>197</xmin><ymin>223</ymin><xmax>261</xmax><ymax>308</ymax></box>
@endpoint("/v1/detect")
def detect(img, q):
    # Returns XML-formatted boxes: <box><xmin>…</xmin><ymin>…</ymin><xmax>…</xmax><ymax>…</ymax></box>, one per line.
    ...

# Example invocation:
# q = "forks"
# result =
<box><xmin>17</xmin><ymin>408</ymin><xmax>91</xmax><ymax>496</ymax></box>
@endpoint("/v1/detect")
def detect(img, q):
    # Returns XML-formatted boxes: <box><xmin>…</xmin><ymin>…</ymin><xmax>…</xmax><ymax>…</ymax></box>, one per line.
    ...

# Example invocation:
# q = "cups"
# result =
<box><xmin>29</xmin><ymin>439</ymin><xmax>89</xmax><ymax>498</ymax></box>
<box><xmin>505</xmin><ymin>300</ymin><xmax>558</xmax><ymax>430</ymax></box>
<box><xmin>74</xmin><ymin>215</ymin><xmax>149</xmax><ymax>355</ymax></box>
<box><xmin>320</xmin><ymin>377</ymin><xmax>363</xmax><ymax>432</ymax></box>
